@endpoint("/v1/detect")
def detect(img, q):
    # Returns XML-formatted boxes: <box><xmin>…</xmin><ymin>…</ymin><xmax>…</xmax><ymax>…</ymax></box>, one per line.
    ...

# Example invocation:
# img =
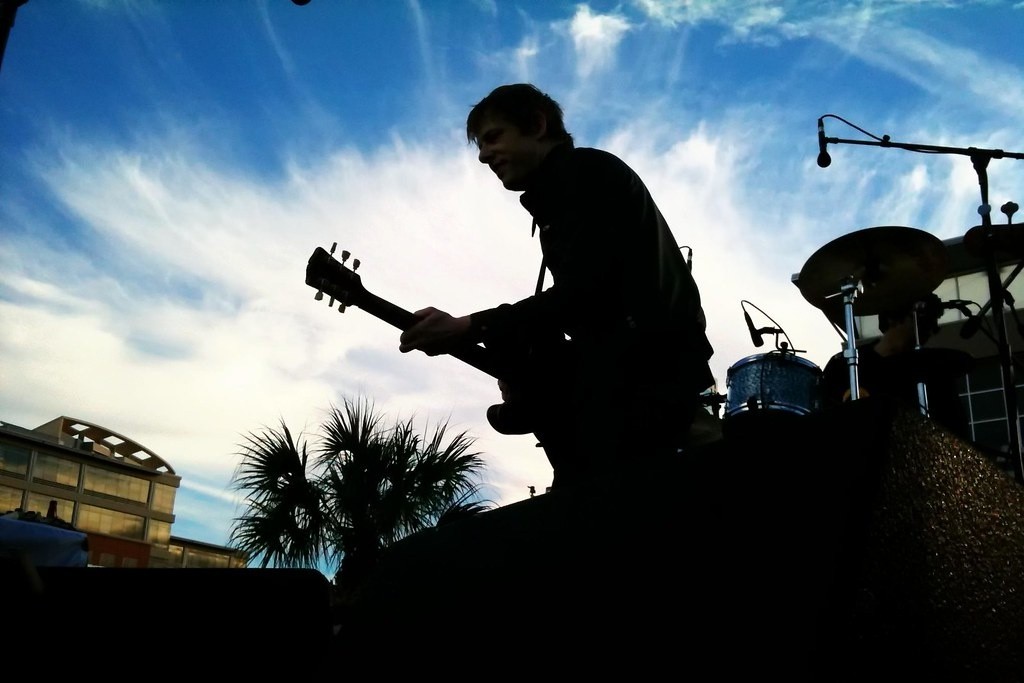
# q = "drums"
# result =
<box><xmin>726</xmin><ymin>352</ymin><xmax>825</xmax><ymax>418</ymax></box>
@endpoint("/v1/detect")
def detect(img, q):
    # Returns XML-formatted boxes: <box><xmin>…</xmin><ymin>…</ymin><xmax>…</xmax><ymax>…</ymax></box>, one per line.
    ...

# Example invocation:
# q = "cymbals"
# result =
<box><xmin>798</xmin><ymin>224</ymin><xmax>948</xmax><ymax>318</ymax></box>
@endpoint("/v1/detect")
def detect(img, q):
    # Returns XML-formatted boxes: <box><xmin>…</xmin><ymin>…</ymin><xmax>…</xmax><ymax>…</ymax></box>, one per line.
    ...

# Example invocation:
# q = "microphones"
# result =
<box><xmin>817</xmin><ymin>118</ymin><xmax>831</xmax><ymax>168</ymax></box>
<box><xmin>744</xmin><ymin>311</ymin><xmax>764</xmax><ymax>347</ymax></box>
<box><xmin>916</xmin><ymin>299</ymin><xmax>972</xmax><ymax>312</ymax></box>
<box><xmin>687</xmin><ymin>249</ymin><xmax>692</xmax><ymax>272</ymax></box>
<box><xmin>959</xmin><ymin>262</ymin><xmax>1024</xmax><ymax>339</ymax></box>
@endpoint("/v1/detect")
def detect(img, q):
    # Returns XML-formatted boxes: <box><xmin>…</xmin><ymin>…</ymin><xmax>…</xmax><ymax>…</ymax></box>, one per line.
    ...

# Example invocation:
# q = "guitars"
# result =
<box><xmin>302</xmin><ymin>240</ymin><xmax>567</xmax><ymax>436</ymax></box>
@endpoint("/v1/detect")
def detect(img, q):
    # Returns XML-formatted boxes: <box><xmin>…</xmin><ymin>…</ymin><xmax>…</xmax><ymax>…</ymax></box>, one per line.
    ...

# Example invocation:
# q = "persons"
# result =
<box><xmin>401</xmin><ymin>84</ymin><xmax>717</xmax><ymax>492</ymax></box>
<box><xmin>821</xmin><ymin>290</ymin><xmax>957</xmax><ymax>432</ymax></box>
